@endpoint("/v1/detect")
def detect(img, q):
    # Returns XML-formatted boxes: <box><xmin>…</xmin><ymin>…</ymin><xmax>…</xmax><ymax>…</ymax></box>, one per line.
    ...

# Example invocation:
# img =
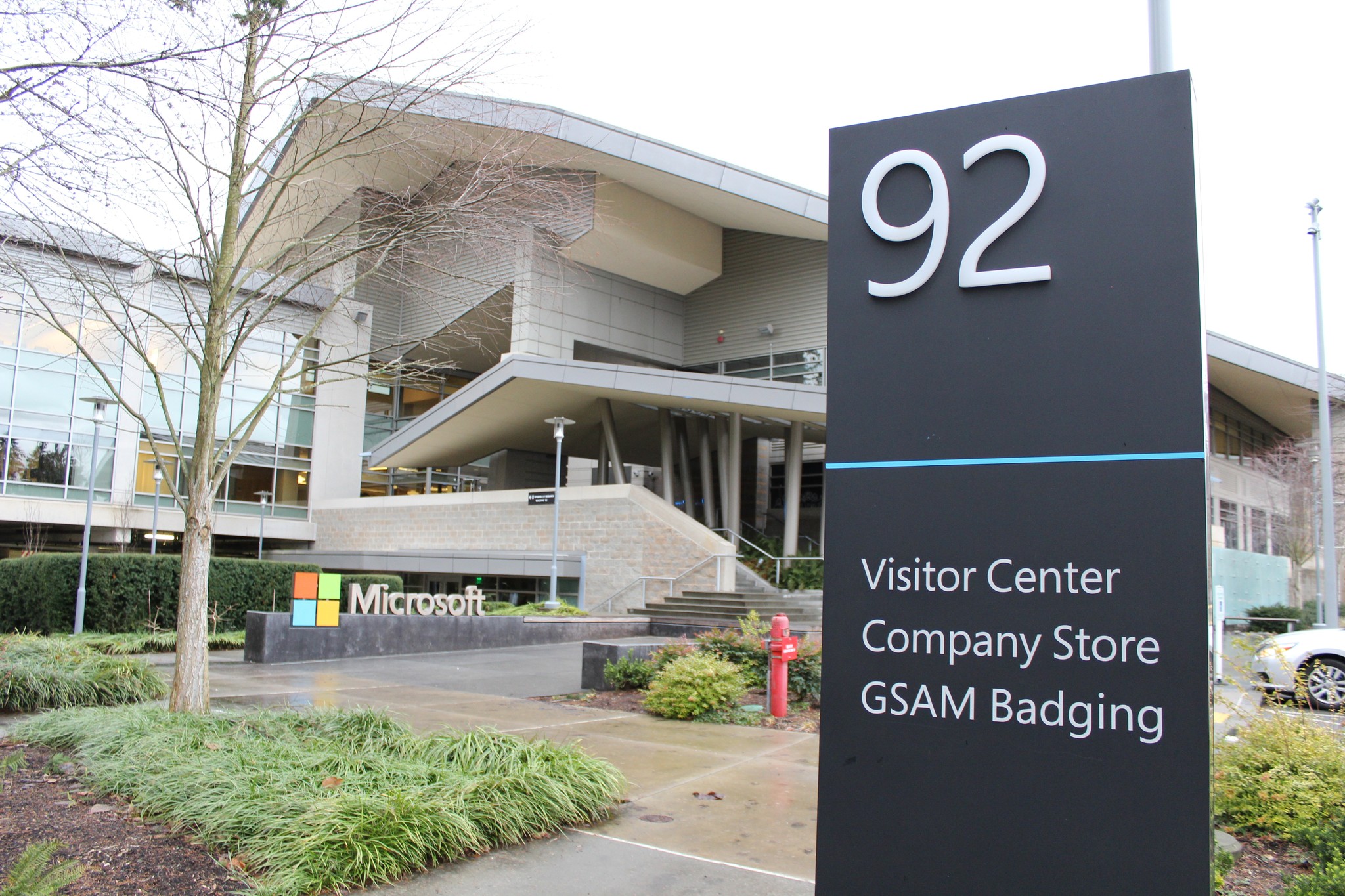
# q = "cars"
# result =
<box><xmin>1252</xmin><ymin>628</ymin><xmax>1345</xmax><ymax>711</ymax></box>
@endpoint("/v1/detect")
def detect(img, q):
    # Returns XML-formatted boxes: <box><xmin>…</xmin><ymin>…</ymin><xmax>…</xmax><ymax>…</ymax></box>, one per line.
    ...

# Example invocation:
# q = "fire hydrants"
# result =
<box><xmin>761</xmin><ymin>613</ymin><xmax>798</xmax><ymax>718</ymax></box>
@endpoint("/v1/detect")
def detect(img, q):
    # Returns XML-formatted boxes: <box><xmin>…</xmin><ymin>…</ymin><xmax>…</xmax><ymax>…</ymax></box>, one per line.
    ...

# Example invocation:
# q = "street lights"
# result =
<box><xmin>545</xmin><ymin>417</ymin><xmax>576</xmax><ymax>609</ymax></box>
<box><xmin>141</xmin><ymin>457</ymin><xmax>174</xmax><ymax>555</ymax></box>
<box><xmin>254</xmin><ymin>491</ymin><xmax>273</xmax><ymax>559</ymax></box>
<box><xmin>73</xmin><ymin>396</ymin><xmax>120</xmax><ymax>633</ymax></box>
<box><xmin>1294</xmin><ymin>441</ymin><xmax>1326</xmax><ymax>630</ymax></box>
